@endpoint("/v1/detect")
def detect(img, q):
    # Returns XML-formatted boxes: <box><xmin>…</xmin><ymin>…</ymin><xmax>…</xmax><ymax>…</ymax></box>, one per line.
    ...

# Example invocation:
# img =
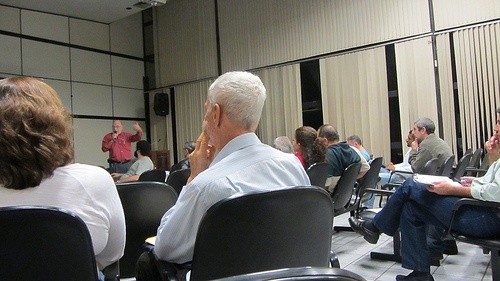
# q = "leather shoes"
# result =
<box><xmin>396</xmin><ymin>273</ymin><xmax>434</xmax><ymax>281</ymax></box>
<box><xmin>349</xmin><ymin>216</ymin><xmax>380</xmax><ymax>245</ymax></box>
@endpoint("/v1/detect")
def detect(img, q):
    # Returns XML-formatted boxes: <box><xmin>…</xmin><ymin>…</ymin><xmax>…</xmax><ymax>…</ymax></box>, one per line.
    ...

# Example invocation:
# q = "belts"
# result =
<box><xmin>114</xmin><ymin>160</ymin><xmax>131</xmax><ymax>164</ymax></box>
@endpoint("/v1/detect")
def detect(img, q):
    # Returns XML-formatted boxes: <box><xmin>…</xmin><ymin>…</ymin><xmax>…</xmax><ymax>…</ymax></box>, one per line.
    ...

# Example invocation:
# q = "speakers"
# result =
<box><xmin>154</xmin><ymin>93</ymin><xmax>169</xmax><ymax>116</ymax></box>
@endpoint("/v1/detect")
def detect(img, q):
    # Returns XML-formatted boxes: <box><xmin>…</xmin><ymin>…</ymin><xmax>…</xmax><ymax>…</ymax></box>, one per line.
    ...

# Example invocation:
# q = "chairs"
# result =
<box><xmin>115</xmin><ymin>181</ymin><xmax>177</xmax><ymax>281</ymax></box>
<box><xmin>167</xmin><ymin>168</ymin><xmax>191</xmax><ymax>195</ymax></box>
<box><xmin>306</xmin><ymin>149</ymin><xmax>500</xmax><ymax>281</ymax></box>
<box><xmin>138</xmin><ymin>168</ymin><xmax>166</xmax><ymax>182</ymax></box>
<box><xmin>141</xmin><ymin>185</ymin><xmax>340</xmax><ymax>281</ymax></box>
<box><xmin>0</xmin><ymin>206</ymin><xmax>121</xmax><ymax>281</ymax></box>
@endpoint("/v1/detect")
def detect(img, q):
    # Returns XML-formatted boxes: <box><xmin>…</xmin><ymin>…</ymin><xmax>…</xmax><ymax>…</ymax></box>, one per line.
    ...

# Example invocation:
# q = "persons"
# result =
<box><xmin>111</xmin><ymin>140</ymin><xmax>171</xmax><ymax>184</ymax></box>
<box><xmin>135</xmin><ymin>70</ymin><xmax>311</xmax><ymax>281</ymax></box>
<box><xmin>0</xmin><ymin>76</ymin><xmax>126</xmax><ymax>281</ymax></box>
<box><xmin>273</xmin><ymin>135</ymin><xmax>298</xmax><ymax>157</ymax></box>
<box><xmin>349</xmin><ymin>109</ymin><xmax>500</xmax><ymax>281</ymax></box>
<box><xmin>101</xmin><ymin>120</ymin><xmax>143</xmax><ymax>174</ymax></box>
<box><xmin>426</xmin><ymin>225</ymin><xmax>458</xmax><ymax>267</ymax></box>
<box><xmin>360</xmin><ymin>117</ymin><xmax>454</xmax><ymax>209</ymax></box>
<box><xmin>182</xmin><ymin>144</ymin><xmax>195</xmax><ymax>168</ymax></box>
<box><xmin>345</xmin><ymin>135</ymin><xmax>371</xmax><ymax>162</ymax></box>
<box><xmin>317</xmin><ymin>124</ymin><xmax>370</xmax><ymax>193</ymax></box>
<box><xmin>291</xmin><ymin>126</ymin><xmax>327</xmax><ymax>171</ymax></box>
